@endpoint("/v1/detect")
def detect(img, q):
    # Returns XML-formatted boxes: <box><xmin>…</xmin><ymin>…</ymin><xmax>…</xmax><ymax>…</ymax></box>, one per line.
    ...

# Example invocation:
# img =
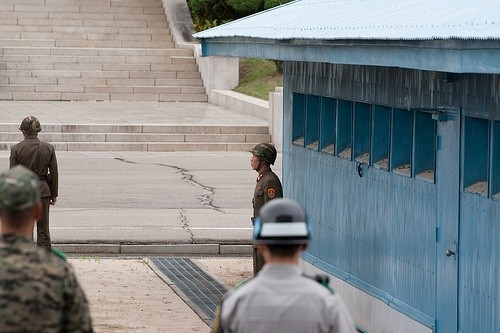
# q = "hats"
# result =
<box><xmin>0</xmin><ymin>164</ymin><xmax>42</xmax><ymax>214</ymax></box>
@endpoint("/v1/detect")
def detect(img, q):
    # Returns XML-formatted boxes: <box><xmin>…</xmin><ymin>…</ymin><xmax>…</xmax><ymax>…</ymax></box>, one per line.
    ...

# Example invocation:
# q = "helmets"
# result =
<box><xmin>249</xmin><ymin>143</ymin><xmax>277</xmax><ymax>166</ymax></box>
<box><xmin>251</xmin><ymin>197</ymin><xmax>313</xmax><ymax>246</ymax></box>
<box><xmin>18</xmin><ymin>115</ymin><xmax>42</xmax><ymax>135</ymax></box>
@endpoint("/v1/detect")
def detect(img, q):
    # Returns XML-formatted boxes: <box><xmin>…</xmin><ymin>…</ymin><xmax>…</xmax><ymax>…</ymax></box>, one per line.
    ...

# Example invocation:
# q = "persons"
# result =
<box><xmin>209</xmin><ymin>196</ymin><xmax>358</xmax><ymax>332</ymax></box>
<box><xmin>1</xmin><ymin>162</ymin><xmax>96</xmax><ymax>333</ymax></box>
<box><xmin>247</xmin><ymin>142</ymin><xmax>284</xmax><ymax>278</ymax></box>
<box><xmin>9</xmin><ymin>114</ymin><xmax>59</xmax><ymax>251</ymax></box>
